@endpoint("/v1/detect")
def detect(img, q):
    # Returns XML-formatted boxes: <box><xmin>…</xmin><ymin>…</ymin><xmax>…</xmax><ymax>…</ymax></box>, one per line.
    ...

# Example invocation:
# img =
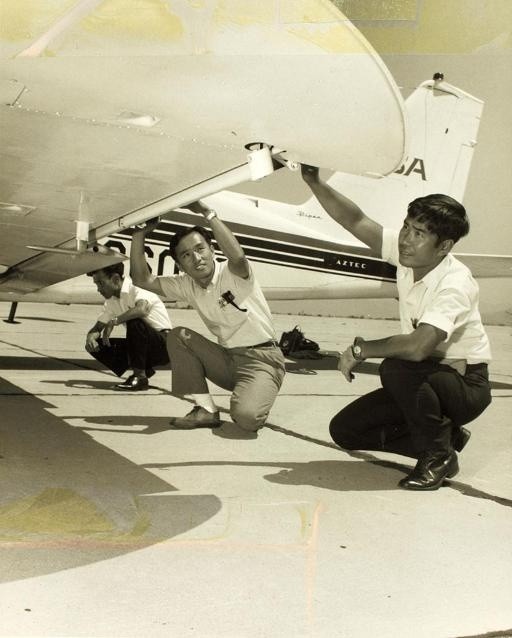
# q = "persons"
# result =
<box><xmin>301</xmin><ymin>161</ymin><xmax>493</xmax><ymax>492</ymax></box>
<box><xmin>84</xmin><ymin>262</ymin><xmax>171</xmax><ymax>392</ymax></box>
<box><xmin>128</xmin><ymin>200</ymin><xmax>286</xmax><ymax>435</ymax></box>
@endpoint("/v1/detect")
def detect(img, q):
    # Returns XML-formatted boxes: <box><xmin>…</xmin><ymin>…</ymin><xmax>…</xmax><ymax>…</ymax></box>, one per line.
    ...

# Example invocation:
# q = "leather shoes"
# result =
<box><xmin>397</xmin><ymin>448</ymin><xmax>459</xmax><ymax>491</ymax></box>
<box><xmin>145</xmin><ymin>366</ymin><xmax>155</xmax><ymax>377</ymax></box>
<box><xmin>452</xmin><ymin>425</ymin><xmax>472</xmax><ymax>454</ymax></box>
<box><xmin>114</xmin><ymin>374</ymin><xmax>148</xmax><ymax>391</ymax></box>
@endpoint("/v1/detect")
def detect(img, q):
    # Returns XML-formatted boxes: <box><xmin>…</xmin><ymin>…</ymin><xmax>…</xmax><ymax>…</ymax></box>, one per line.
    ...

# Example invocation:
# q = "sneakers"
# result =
<box><xmin>169</xmin><ymin>405</ymin><xmax>221</xmax><ymax>429</ymax></box>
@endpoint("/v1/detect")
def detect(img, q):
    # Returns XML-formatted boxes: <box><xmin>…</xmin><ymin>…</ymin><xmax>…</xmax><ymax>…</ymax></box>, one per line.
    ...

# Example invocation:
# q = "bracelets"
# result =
<box><xmin>112</xmin><ymin>316</ymin><xmax>117</xmax><ymax>325</ymax></box>
<box><xmin>206</xmin><ymin>209</ymin><xmax>216</xmax><ymax>221</ymax></box>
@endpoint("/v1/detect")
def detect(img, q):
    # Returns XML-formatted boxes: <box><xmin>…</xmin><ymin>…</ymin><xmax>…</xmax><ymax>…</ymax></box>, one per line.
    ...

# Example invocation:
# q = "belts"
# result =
<box><xmin>247</xmin><ymin>341</ymin><xmax>280</xmax><ymax>347</ymax></box>
<box><xmin>161</xmin><ymin>328</ymin><xmax>171</xmax><ymax>333</ymax></box>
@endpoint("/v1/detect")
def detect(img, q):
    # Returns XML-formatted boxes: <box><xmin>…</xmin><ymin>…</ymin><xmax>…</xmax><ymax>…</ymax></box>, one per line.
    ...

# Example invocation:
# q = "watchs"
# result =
<box><xmin>352</xmin><ymin>337</ymin><xmax>363</xmax><ymax>360</ymax></box>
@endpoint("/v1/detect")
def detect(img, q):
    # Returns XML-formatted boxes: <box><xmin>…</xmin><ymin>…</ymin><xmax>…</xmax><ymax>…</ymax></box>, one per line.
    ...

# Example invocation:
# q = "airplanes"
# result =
<box><xmin>0</xmin><ymin>0</ymin><xmax>511</xmax><ymax>328</ymax></box>
